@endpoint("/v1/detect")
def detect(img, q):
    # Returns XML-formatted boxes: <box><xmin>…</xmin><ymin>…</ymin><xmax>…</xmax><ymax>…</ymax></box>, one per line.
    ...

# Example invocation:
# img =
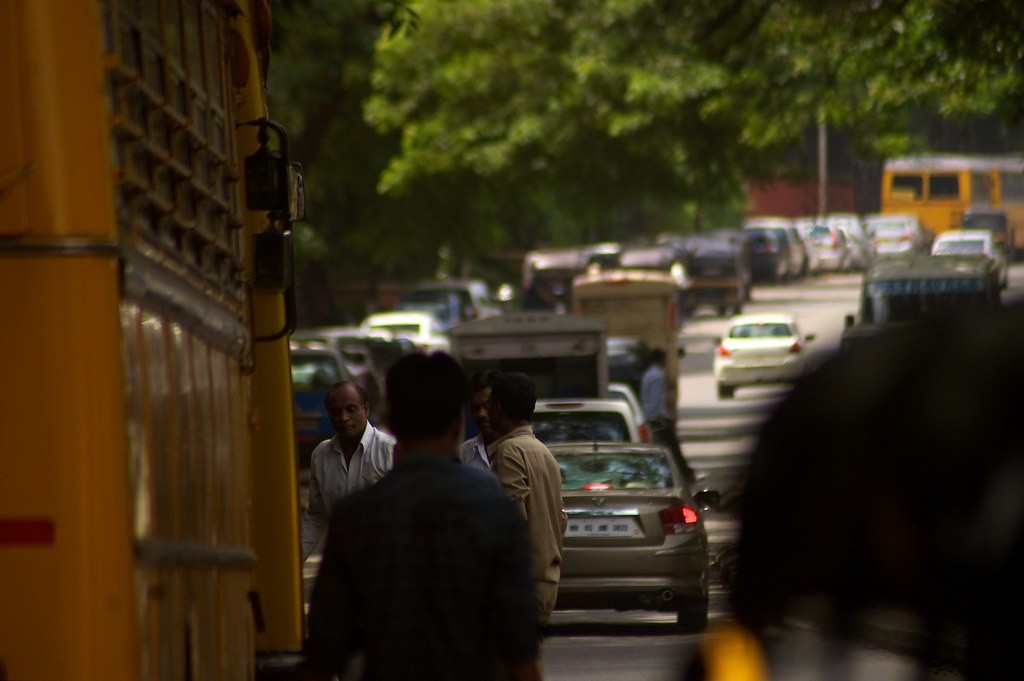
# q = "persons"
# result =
<box><xmin>306</xmin><ymin>381</ymin><xmax>396</xmax><ymax>529</ymax></box>
<box><xmin>637</xmin><ymin>346</ymin><xmax>694</xmax><ymax>479</ymax></box>
<box><xmin>457</xmin><ymin>368</ymin><xmax>502</xmax><ymax>471</ymax></box>
<box><xmin>299</xmin><ymin>351</ymin><xmax>544</xmax><ymax>681</ymax></box>
<box><xmin>487</xmin><ymin>372</ymin><xmax>568</xmax><ymax>681</ymax></box>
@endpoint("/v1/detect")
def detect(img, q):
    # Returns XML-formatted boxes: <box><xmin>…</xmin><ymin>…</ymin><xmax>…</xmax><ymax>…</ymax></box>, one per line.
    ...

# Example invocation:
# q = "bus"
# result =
<box><xmin>882</xmin><ymin>153</ymin><xmax>1024</xmax><ymax>258</ymax></box>
<box><xmin>1</xmin><ymin>0</ymin><xmax>307</xmax><ymax>681</ymax></box>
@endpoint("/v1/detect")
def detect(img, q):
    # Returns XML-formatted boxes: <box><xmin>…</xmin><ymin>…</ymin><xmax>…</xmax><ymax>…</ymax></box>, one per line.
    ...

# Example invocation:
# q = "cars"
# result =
<box><xmin>711</xmin><ymin>313</ymin><xmax>816</xmax><ymax>398</ymax></box>
<box><xmin>296</xmin><ymin>205</ymin><xmax>1024</xmax><ymax>681</ymax></box>
<box><xmin>536</xmin><ymin>400</ymin><xmax>648</xmax><ymax>449</ymax></box>
<box><xmin>547</xmin><ymin>442</ymin><xmax>721</xmax><ymax>636</ymax></box>
